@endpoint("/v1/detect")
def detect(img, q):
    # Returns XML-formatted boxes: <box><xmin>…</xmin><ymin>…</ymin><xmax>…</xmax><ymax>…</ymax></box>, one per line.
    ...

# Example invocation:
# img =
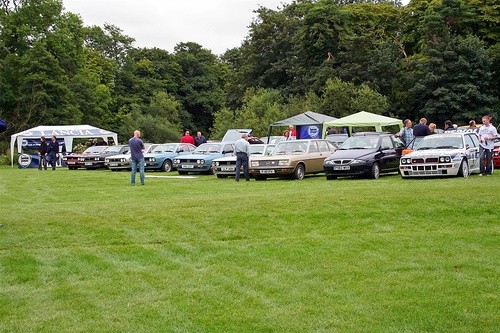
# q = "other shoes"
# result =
<box><xmin>246</xmin><ymin>179</ymin><xmax>250</xmax><ymax>181</ymax></box>
<box><xmin>236</xmin><ymin>179</ymin><xmax>239</xmax><ymax>181</ymax></box>
<box><xmin>486</xmin><ymin>174</ymin><xmax>491</xmax><ymax>176</ymax></box>
<box><xmin>478</xmin><ymin>173</ymin><xmax>484</xmax><ymax>176</ymax></box>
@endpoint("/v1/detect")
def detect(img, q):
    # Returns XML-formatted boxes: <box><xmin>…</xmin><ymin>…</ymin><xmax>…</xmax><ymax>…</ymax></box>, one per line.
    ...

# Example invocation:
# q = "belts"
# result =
<box><xmin>237</xmin><ymin>152</ymin><xmax>245</xmax><ymax>154</ymax></box>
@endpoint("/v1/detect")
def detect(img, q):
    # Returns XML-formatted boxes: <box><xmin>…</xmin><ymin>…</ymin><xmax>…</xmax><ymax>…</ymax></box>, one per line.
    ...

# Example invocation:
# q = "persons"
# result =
<box><xmin>280</xmin><ymin>125</ymin><xmax>296</xmax><ymax>142</ymax></box>
<box><xmin>45</xmin><ymin>135</ymin><xmax>59</xmax><ymax>171</ymax></box>
<box><xmin>194</xmin><ymin>131</ymin><xmax>207</xmax><ymax>147</ymax></box>
<box><xmin>395</xmin><ymin>116</ymin><xmax>498</xmax><ymax>176</ymax></box>
<box><xmin>232</xmin><ymin>134</ymin><xmax>251</xmax><ymax>181</ymax></box>
<box><xmin>108</xmin><ymin>143</ymin><xmax>112</xmax><ymax>146</ymax></box>
<box><xmin>129</xmin><ymin>130</ymin><xmax>145</xmax><ymax>186</ymax></box>
<box><xmin>108</xmin><ymin>137</ymin><xmax>114</xmax><ymax>145</ymax></box>
<box><xmin>38</xmin><ymin>136</ymin><xmax>47</xmax><ymax>170</ymax></box>
<box><xmin>180</xmin><ymin>131</ymin><xmax>195</xmax><ymax>144</ymax></box>
<box><xmin>87</xmin><ymin>137</ymin><xmax>107</xmax><ymax>147</ymax></box>
<box><xmin>327</xmin><ymin>126</ymin><xmax>355</xmax><ymax>135</ymax></box>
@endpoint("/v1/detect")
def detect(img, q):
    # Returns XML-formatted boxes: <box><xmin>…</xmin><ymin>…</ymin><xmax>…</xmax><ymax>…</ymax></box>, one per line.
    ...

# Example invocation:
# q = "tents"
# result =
<box><xmin>322</xmin><ymin>111</ymin><xmax>404</xmax><ymax>140</ymax></box>
<box><xmin>11</xmin><ymin>125</ymin><xmax>119</xmax><ymax>170</ymax></box>
<box><xmin>267</xmin><ymin>111</ymin><xmax>337</xmax><ymax>144</ymax></box>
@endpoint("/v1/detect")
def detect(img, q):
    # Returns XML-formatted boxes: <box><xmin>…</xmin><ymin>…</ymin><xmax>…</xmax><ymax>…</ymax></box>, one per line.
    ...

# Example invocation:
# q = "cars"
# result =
<box><xmin>323</xmin><ymin>132</ymin><xmax>408</xmax><ymax>180</ymax></box>
<box><xmin>399</xmin><ymin>133</ymin><xmax>485</xmax><ymax>178</ymax></box>
<box><xmin>485</xmin><ymin>142</ymin><xmax>500</xmax><ymax>169</ymax></box>
<box><xmin>130</xmin><ymin>143</ymin><xmax>197</xmax><ymax>172</ymax></box>
<box><xmin>78</xmin><ymin>145</ymin><xmax>131</xmax><ymax>170</ymax></box>
<box><xmin>213</xmin><ymin>135</ymin><xmax>285</xmax><ymax>178</ymax></box>
<box><xmin>173</xmin><ymin>128</ymin><xmax>252</xmax><ymax>175</ymax></box>
<box><xmin>251</xmin><ymin>138</ymin><xmax>339</xmax><ymax>180</ymax></box>
<box><xmin>104</xmin><ymin>143</ymin><xmax>160</xmax><ymax>172</ymax></box>
<box><xmin>63</xmin><ymin>144</ymin><xmax>109</xmax><ymax>171</ymax></box>
